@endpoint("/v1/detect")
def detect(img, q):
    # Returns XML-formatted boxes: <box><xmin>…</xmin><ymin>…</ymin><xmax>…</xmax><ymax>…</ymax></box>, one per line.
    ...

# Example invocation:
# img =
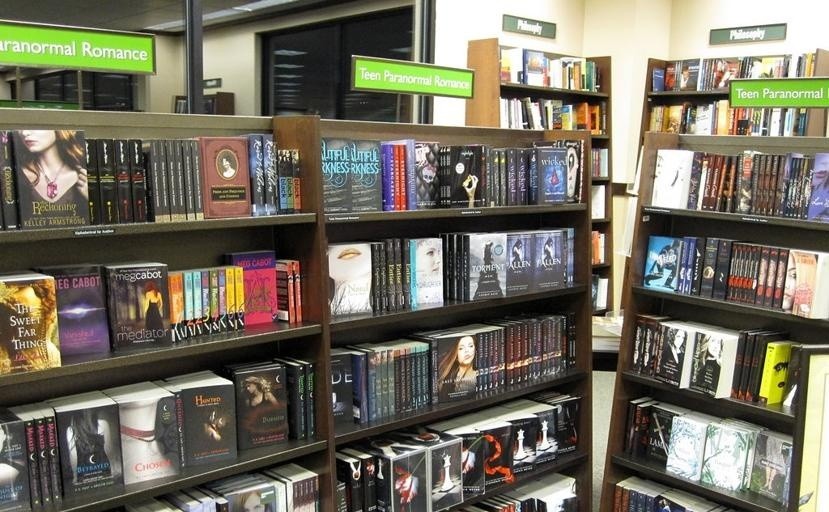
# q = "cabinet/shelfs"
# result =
<box><xmin>0</xmin><ymin>104</ymin><xmax>594</xmax><ymax>512</ymax></box>
<box><xmin>466</xmin><ymin>38</ymin><xmax>615</xmax><ymax>316</ymax></box>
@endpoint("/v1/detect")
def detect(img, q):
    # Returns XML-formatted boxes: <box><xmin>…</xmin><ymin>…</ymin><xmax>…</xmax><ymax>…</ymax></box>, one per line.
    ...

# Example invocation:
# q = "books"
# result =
<box><xmin>624</xmin><ymin>397</ymin><xmax>795</xmax><ymax>506</ymax></box>
<box><xmin>0</xmin><ymin>128</ymin><xmax>302</xmax><ymax>230</ymax></box>
<box><xmin>335</xmin><ymin>392</ymin><xmax>581</xmax><ymax>512</ymax></box>
<box><xmin>615</xmin><ymin>475</ymin><xmax>730</xmax><ymax>512</ymax></box>
<box><xmin>649</xmin><ymin>53</ymin><xmax>816</xmax><ymax>93</ymax></box>
<box><xmin>498</xmin><ymin>46</ymin><xmax>586</xmax><ymax>133</ymax></box>
<box><xmin>329</xmin><ymin>228</ymin><xmax>575</xmax><ymax>318</ymax></box>
<box><xmin>632</xmin><ymin>313</ymin><xmax>799</xmax><ymax>405</ymax></box>
<box><xmin>332</xmin><ymin>310</ymin><xmax>576</xmax><ymax>427</ymax></box>
<box><xmin>653</xmin><ymin>149</ymin><xmax>829</xmax><ymax>221</ymax></box>
<box><xmin>586</xmin><ymin>60</ymin><xmax>609</xmax><ymax>309</ymax></box>
<box><xmin>650</xmin><ymin>99</ymin><xmax>808</xmax><ymax>137</ymax></box>
<box><xmin>321</xmin><ymin>135</ymin><xmax>585</xmax><ymax>213</ymax></box>
<box><xmin>643</xmin><ymin>235</ymin><xmax>829</xmax><ymax>320</ymax></box>
<box><xmin>0</xmin><ymin>251</ymin><xmax>302</xmax><ymax>372</ymax></box>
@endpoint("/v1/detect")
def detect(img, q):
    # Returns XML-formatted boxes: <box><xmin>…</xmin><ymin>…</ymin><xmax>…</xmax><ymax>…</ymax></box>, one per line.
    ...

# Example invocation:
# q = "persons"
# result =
<box><xmin>0</xmin><ymin>355</ymin><xmax>320</xmax><ymax>512</ymax></box>
